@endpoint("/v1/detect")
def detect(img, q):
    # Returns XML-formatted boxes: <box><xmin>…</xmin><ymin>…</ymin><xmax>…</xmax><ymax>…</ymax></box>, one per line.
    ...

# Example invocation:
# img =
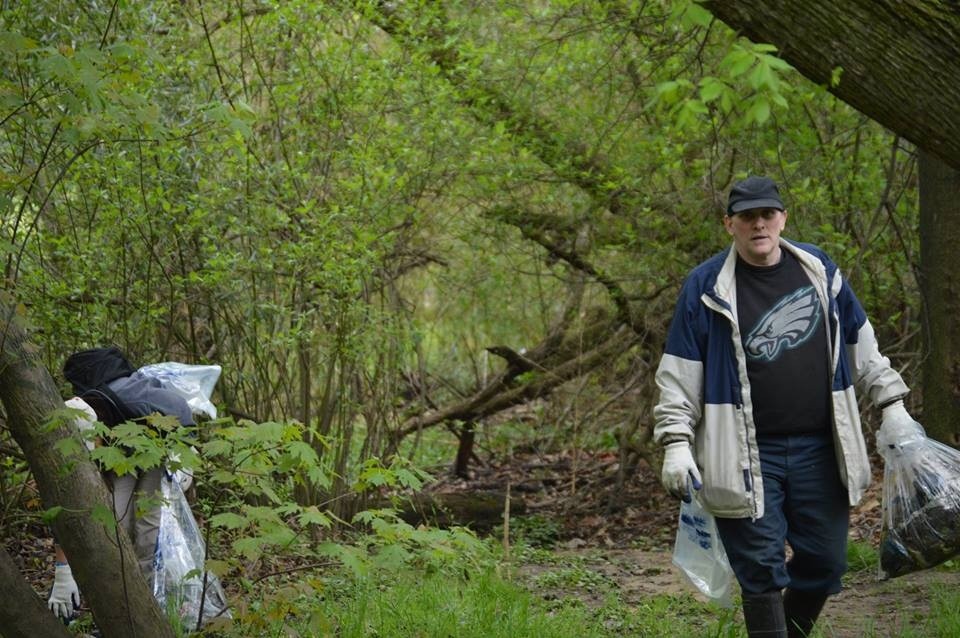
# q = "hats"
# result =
<box><xmin>728</xmin><ymin>176</ymin><xmax>784</xmax><ymax>212</ymax></box>
<box><xmin>62</xmin><ymin>398</ymin><xmax>97</xmax><ymax>453</ymax></box>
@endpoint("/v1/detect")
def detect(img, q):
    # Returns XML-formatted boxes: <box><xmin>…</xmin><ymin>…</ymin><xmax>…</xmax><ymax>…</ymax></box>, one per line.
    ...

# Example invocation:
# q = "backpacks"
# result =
<box><xmin>63</xmin><ymin>347</ymin><xmax>136</xmax><ymax>423</ymax></box>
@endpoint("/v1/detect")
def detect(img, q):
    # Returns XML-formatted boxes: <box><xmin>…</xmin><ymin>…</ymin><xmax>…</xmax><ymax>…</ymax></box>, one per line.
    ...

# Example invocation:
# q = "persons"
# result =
<box><xmin>653</xmin><ymin>174</ymin><xmax>916</xmax><ymax>638</ymax></box>
<box><xmin>47</xmin><ymin>372</ymin><xmax>198</xmax><ymax>619</ymax></box>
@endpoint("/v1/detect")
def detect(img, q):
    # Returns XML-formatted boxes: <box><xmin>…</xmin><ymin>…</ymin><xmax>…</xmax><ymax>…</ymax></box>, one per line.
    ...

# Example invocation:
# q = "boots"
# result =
<box><xmin>741</xmin><ymin>592</ymin><xmax>788</xmax><ymax>638</ymax></box>
<box><xmin>785</xmin><ymin>588</ymin><xmax>827</xmax><ymax>638</ymax></box>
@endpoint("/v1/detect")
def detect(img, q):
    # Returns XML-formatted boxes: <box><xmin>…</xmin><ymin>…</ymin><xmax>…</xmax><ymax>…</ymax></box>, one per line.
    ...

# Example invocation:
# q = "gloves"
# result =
<box><xmin>661</xmin><ymin>441</ymin><xmax>702</xmax><ymax>503</ymax></box>
<box><xmin>165</xmin><ymin>449</ymin><xmax>181</xmax><ymax>484</ymax></box>
<box><xmin>48</xmin><ymin>563</ymin><xmax>80</xmax><ymax>618</ymax></box>
<box><xmin>879</xmin><ymin>399</ymin><xmax>916</xmax><ymax>430</ymax></box>
